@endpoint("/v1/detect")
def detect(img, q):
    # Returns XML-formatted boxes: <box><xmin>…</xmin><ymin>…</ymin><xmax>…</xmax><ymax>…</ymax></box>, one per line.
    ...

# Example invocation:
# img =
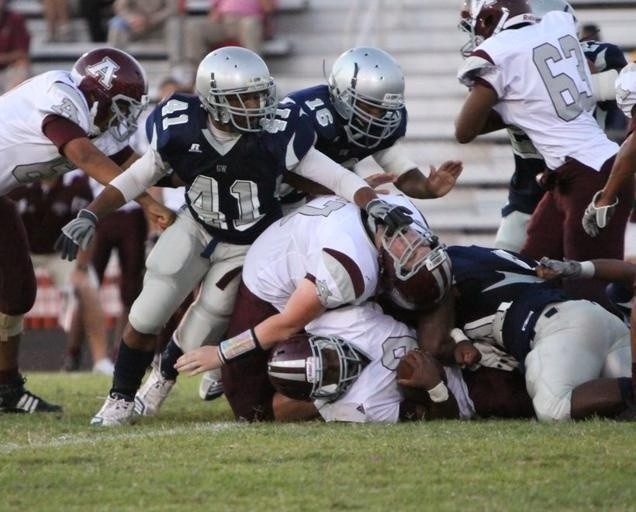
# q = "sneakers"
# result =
<box><xmin>136</xmin><ymin>367</ymin><xmax>175</xmax><ymax>418</ymax></box>
<box><xmin>87</xmin><ymin>394</ymin><xmax>135</xmax><ymax>431</ymax></box>
<box><xmin>0</xmin><ymin>374</ymin><xmax>62</xmax><ymax>414</ymax></box>
<box><xmin>199</xmin><ymin>374</ymin><xmax>222</xmax><ymax>400</ymax></box>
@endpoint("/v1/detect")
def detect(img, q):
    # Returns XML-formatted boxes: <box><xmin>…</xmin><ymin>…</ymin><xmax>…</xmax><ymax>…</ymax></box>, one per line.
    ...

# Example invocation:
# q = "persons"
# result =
<box><xmin>0</xmin><ymin>48</ymin><xmax>175</xmax><ymax>413</ymax></box>
<box><xmin>39</xmin><ymin>0</ymin><xmax>115</xmax><ymax>43</ymax></box>
<box><xmin>175</xmin><ymin>192</ymin><xmax>454</xmax><ymax>424</ymax></box>
<box><xmin>0</xmin><ymin>0</ymin><xmax>31</xmax><ymax>92</ymax></box>
<box><xmin>536</xmin><ymin>254</ymin><xmax>636</xmax><ymax>328</ymax></box>
<box><xmin>200</xmin><ymin>45</ymin><xmax>462</xmax><ymax>401</ymax></box>
<box><xmin>495</xmin><ymin>1</ymin><xmax>630</xmax><ymax>257</ymax></box>
<box><xmin>456</xmin><ymin>0</ymin><xmax>630</xmax><ymax>262</ymax></box>
<box><xmin>192</xmin><ymin>0</ymin><xmax>278</xmax><ymax>61</ymax></box>
<box><xmin>58</xmin><ymin>47</ymin><xmax>412</xmax><ymax>431</ymax></box>
<box><xmin>109</xmin><ymin>0</ymin><xmax>184</xmax><ymax>64</ymax></box>
<box><xmin>447</xmin><ymin>244</ymin><xmax>634</xmax><ymax>420</ymax></box>
<box><xmin>273</xmin><ymin>303</ymin><xmax>534</xmax><ymax>421</ymax></box>
<box><xmin>64</xmin><ymin>163</ymin><xmax>147</xmax><ymax>372</ymax></box>
<box><xmin>580</xmin><ymin>60</ymin><xmax>636</xmax><ymax>236</ymax></box>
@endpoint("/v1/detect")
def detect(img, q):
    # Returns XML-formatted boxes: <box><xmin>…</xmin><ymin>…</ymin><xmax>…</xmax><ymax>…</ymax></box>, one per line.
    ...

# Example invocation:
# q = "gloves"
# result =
<box><xmin>54</xmin><ymin>209</ymin><xmax>99</xmax><ymax>261</ymax></box>
<box><xmin>582</xmin><ymin>190</ymin><xmax>619</xmax><ymax>237</ymax></box>
<box><xmin>535</xmin><ymin>257</ymin><xmax>582</xmax><ymax>286</ymax></box>
<box><xmin>366</xmin><ymin>199</ymin><xmax>414</xmax><ymax>233</ymax></box>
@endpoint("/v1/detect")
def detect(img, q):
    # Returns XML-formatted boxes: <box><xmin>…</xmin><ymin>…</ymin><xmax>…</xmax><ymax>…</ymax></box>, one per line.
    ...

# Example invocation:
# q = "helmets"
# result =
<box><xmin>70</xmin><ymin>48</ymin><xmax>149</xmax><ymax>142</ymax></box>
<box><xmin>194</xmin><ymin>46</ymin><xmax>278</xmax><ymax>133</ymax></box>
<box><xmin>328</xmin><ymin>47</ymin><xmax>405</xmax><ymax>148</ymax></box>
<box><xmin>458</xmin><ymin>0</ymin><xmax>536</xmax><ymax>49</ymax></box>
<box><xmin>615</xmin><ymin>62</ymin><xmax>635</xmax><ymax>118</ymax></box>
<box><xmin>381</xmin><ymin>221</ymin><xmax>452</xmax><ymax>312</ymax></box>
<box><xmin>268</xmin><ymin>334</ymin><xmax>362</xmax><ymax>400</ymax></box>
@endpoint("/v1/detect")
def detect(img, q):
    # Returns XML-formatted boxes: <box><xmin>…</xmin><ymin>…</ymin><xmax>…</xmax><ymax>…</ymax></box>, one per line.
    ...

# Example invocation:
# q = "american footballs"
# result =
<box><xmin>397</xmin><ymin>354</ymin><xmax>448</xmax><ymax>404</ymax></box>
